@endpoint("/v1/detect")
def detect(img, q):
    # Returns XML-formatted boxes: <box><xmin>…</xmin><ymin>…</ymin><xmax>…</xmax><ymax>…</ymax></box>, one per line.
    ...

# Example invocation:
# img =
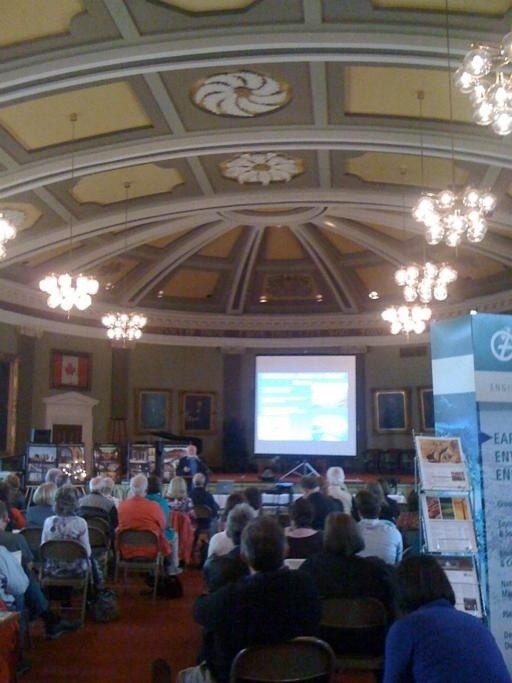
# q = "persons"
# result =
<box><xmin>297</xmin><ymin>512</ymin><xmax>399</xmax><ymax>660</ymax></box>
<box><xmin>151</xmin><ymin>516</ymin><xmax>321</xmax><ymax>683</ymax></box>
<box><xmin>144</xmin><ymin>397</ymin><xmax>161</xmax><ymax>427</ymax></box>
<box><xmin>189</xmin><ymin>400</ymin><xmax>204</xmax><ymax>429</ymax></box>
<box><xmin>381</xmin><ymin>554</ymin><xmax>512</xmax><ymax>683</ymax></box>
<box><xmin>1</xmin><ymin>445</ymin><xmax>221</xmax><ymax>640</ymax></box>
<box><xmin>202</xmin><ymin>467</ymin><xmax>417</xmax><ymax>589</ymax></box>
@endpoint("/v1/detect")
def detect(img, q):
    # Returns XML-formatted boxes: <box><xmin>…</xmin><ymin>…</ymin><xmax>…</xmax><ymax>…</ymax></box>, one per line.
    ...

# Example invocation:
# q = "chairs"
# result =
<box><xmin>0</xmin><ymin>479</ymin><xmax>417</xmax><ymax>681</ymax></box>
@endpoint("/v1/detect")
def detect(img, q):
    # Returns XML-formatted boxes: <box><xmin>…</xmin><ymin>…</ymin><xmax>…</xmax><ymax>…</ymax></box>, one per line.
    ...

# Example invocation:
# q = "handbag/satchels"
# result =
<box><xmin>89</xmin><ymin>586</ymin><xmax>119</xmax><ymax>625</ymax></box>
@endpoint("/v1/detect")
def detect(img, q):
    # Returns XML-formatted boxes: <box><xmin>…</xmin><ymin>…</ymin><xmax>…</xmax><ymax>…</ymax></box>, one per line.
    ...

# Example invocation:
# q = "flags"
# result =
<box><xmin>55</xmin><ymin>355</ymin><xmax>88</xmax><ymax>386</ymax></box>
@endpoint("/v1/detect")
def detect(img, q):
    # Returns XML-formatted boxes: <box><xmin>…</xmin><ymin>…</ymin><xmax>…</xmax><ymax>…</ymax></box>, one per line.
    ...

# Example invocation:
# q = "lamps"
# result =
<box><xmin>1</xmin><ymin>205</ymin><xmax>18</xmax><ymax>260</ymax></box>
<box><xmin>105</xmin><ymin>178</ymin><xmax>147</xmax><ymax>353</ymax></box>
<box><xmin>410</xmin><ymin>0</ymin><xmax>496</xmax><ymax>252</ymax></box>
<box><xmin>377</xmin><ymin>160</ymin><xmax>431</xmax><ymax>341</ymax></box>
<box><xmin>448</xmin><ymin>28</ymin><xmax>512</xmax><ymax>139</ymax></box>
<box><xmin>394</xmin><ymin>85</ymin><xmax>457</xmax><ymax>307</ymax></box>
<box><xmin>39</xmin><ymin>111</ymin><xmax>102</xmax><ymax>321</ymax></box>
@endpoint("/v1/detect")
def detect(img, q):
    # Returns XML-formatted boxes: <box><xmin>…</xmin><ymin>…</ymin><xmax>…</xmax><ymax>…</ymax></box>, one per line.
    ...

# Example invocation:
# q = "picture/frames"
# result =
<box><xmin>416</xmin><ymin>383</ymin><xmax>436</xmax><ymax>433</ymax></box>
<box><xmin>177</xmin><ymin>387</ymin><xmax>219</xmax><ymax>436</ymax></box>
<box><xmin>49</xmin><ymin>348</ymin><xmax>94</xmax><ymax>392</ymax></box>
<box><xmin>133</xmin><ymin>384</ymin><xmax>173</xmax><ymax>436</ymax></box>
<box><xmin>370</xmin><ymin>386</ymin><xmax>413</xmax><ymax>436</ymax></box>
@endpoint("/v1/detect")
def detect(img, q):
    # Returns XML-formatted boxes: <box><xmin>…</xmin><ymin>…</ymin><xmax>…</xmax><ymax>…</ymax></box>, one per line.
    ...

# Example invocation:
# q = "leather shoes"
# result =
<box><xmin>45</xmin><ymin>619</ymin><xmax>77</xmax><ymax>637</ymax></box>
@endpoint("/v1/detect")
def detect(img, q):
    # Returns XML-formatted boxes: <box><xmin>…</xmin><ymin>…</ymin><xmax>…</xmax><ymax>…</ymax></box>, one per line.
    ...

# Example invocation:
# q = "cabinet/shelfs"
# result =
<box><xmin>24</xmin><ymin>440</ymin><xmax>198</xmax><ymax>491</ymax></box>
